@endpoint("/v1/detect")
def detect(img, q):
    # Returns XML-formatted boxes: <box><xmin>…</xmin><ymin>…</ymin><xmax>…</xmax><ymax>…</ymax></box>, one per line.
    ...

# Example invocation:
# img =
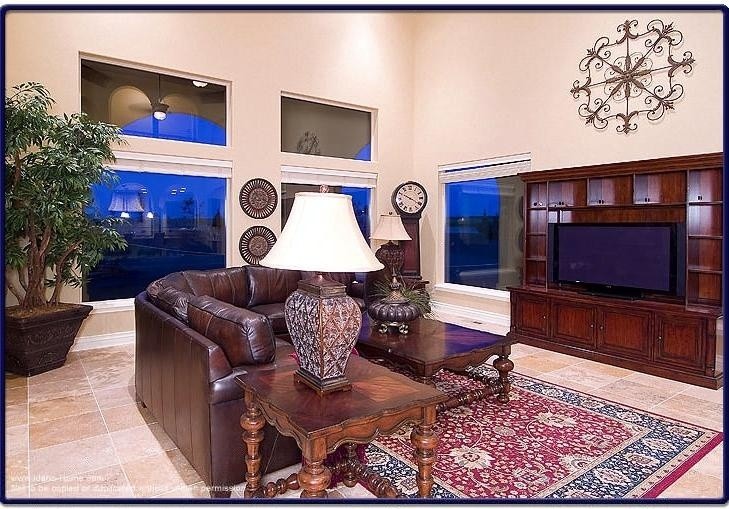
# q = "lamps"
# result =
<box><xmin>369</xmin><ymin>212</ymin><xmax>413</xmax><ymax>278</ymax></box>
<box><xmin>259</xmin><ymin>186</ymin><xmax>385</xmax><ymax>397</ymax></box>
<box><xmin>152</xmin><ymin>101</ymin><xmax>169</xmax><ymax>120</ymax></box>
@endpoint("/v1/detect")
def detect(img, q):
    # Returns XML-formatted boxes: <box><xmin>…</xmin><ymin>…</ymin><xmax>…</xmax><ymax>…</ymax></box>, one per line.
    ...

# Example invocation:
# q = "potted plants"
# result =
<box><xmin>1</xmin><ymin>82</ymin><xmax>130</xmax><ymax>378</ymax></box>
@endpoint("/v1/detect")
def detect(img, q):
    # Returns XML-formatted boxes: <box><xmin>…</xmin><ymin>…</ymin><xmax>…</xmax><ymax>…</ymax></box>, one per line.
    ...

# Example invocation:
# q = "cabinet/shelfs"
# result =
<box><xmin>549</xmin><ymin>300</ymin><xmax>653</xmax><ymax>365</ymax></box>
<box><xmin>516</xmin><ymin>151</ymin><xmax>724</xmax><ymax>307</ymax></box>
<box><xmin>511</xmin><ymin>292</ymin><xmax>549</xmax><ymax>343</ymax></box>
<box><xmin>654</xmin><ymin>314</ymin><xmax>707</xmax><ymax>374</ymax></box>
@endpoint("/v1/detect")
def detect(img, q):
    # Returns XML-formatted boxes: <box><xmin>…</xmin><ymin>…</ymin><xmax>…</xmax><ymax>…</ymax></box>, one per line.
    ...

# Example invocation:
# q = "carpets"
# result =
<box><xmin>343</xmin><ymin>362</ymin><xmax>724</xmax><ymax>499</ymax></box>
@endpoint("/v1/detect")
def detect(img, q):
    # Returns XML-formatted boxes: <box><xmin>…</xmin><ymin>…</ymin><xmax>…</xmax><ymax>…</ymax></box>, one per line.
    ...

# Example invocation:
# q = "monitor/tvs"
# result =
<box><xmin>551</xmin><ymin>223</ymin><xmax>678</xmax><ymax>301</ymax></box>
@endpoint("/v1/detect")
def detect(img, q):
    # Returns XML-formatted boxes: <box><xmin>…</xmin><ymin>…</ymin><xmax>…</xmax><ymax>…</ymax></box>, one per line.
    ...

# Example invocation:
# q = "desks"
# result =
<box><xmin>233</xmin><ymin>355</ymin><xmax>450</xmax><ymax>500</ymax></box>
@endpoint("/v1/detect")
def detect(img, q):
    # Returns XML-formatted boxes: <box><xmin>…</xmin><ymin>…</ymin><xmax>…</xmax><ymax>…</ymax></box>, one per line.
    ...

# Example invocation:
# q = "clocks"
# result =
<box><xmin>391</xmin><ymin>182</ymin><xmax>430</xmax><ymax>279</ymax></box>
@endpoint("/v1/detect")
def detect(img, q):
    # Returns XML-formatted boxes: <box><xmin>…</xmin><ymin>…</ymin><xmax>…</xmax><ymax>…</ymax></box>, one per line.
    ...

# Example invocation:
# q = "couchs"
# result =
<box><xmin>135</xmin><ymin>266</ymin><xmax>365</xmax><ymax>499</ymax></box>
